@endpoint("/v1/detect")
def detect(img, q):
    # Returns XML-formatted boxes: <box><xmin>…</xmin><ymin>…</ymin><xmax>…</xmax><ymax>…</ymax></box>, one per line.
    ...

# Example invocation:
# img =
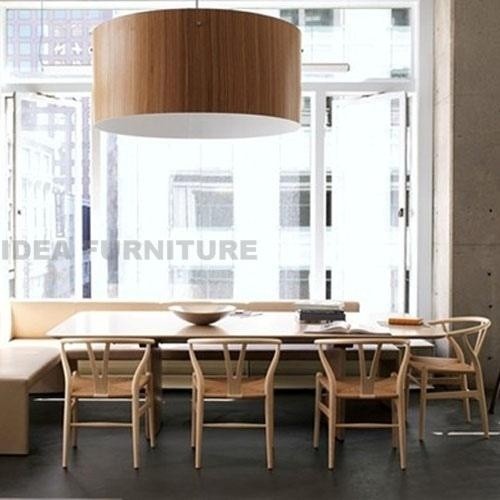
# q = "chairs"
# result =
<box><xmin>186</xmin><ymin>337</ymin><xmax>283</xmax><ymax>470</ymax></box>
<box><xmin>58</xmin><ymin>337</ymin><xmax>159</xmax><ymax>471</ymax></box>
<box><xmin>409</xmin><ymin>314</ymin><xmax>492</xmax><ymax>444</ymax></box>
<box><xmin>314</xmin><ymin>337</ymin><xmax>410</xmax><ymax>470</ymax></box>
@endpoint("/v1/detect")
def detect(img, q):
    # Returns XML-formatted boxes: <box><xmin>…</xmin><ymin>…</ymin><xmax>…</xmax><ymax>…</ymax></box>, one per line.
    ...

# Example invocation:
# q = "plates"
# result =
<box><xmin>167</xmin><ymin>304</ymin><xmax>237</xmax><ymax>326</ymax></box>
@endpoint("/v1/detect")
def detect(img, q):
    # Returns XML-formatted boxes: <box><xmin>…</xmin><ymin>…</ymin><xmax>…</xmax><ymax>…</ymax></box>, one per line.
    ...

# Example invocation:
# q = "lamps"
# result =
<box><xmin>92</xmin><ymin>0</ymin><xmax>302</xmax><ymax>141</ymax></box>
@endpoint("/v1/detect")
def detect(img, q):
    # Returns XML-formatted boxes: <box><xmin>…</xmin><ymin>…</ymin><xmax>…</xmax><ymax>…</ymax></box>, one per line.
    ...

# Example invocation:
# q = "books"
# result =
<box><xmin>389</xmin><ymin>314</ymin><xmax>424</xmax><ymax>325</ymax></box>
<box><xmin>294</xmin><ymin>299</ymin><xmax>393</xmax><ymax>335</ymax></box>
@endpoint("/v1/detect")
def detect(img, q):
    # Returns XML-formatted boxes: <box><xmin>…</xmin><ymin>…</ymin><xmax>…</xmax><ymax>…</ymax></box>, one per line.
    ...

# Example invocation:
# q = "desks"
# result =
<box><xmin>45</xmin><ymin>310</ymin><xmax>449</xmax><ymax>445</ymax></box>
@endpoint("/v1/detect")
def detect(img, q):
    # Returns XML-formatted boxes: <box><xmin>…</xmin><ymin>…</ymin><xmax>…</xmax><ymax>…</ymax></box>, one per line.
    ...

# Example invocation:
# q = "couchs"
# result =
<box><xmin>0</xmin><ymin>298</ymin><xmax>360</xmax><ymax>456</ymax></box>
